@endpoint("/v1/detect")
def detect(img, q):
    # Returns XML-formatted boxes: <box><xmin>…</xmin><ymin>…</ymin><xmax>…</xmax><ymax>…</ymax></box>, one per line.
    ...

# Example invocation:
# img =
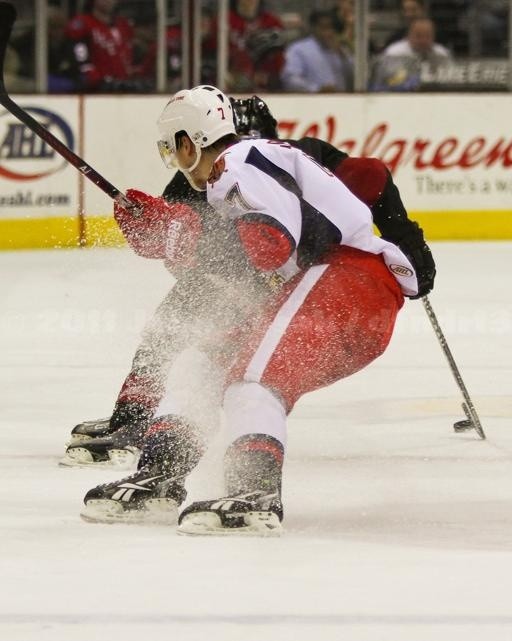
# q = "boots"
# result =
<box><xmin>65</xmin><ymin>419</ymin><xmax>147</xmax><ymax>463</ymax></box>
<box><xmin>71</xmin><ymin>405</ymin><xmax>144</xmax><ymax>436</ymax></box>
<box><xmin>177</xmin><ymin>473</ymin><xmax>284</xmax><ymax>528</ymax></box>
<box><xmin>85</xmin><ymin>452</ymin><xmax>189</xmax><ymax>505</ymax></box>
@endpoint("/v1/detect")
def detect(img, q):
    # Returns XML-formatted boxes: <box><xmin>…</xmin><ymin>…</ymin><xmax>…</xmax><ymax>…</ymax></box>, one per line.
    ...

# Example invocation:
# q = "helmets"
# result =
<box><xmin>156</xmin><ymin>85</ymin><xmax>236</xmax><ymax>175</ymax></box>
<box><xmin>229</xmin><ymin>94</ymin><xmax>279</xmax><ymax>142</ymax></box>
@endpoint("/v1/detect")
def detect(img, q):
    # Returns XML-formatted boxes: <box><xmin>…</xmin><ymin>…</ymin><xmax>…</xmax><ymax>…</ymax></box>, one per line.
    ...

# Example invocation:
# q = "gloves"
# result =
<box><xmin>113</xmin><ymin>188</ymin><xmax>201</xmax><ymax>273</ymax></box>
<box><xmin>379</xmin><ymin>222</ymin><xmax>437</xmax><ymax>300</ymax></box>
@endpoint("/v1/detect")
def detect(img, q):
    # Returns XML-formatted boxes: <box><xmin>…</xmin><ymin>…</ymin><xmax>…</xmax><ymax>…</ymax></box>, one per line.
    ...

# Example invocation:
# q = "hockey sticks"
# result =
<box><xmin>0</xmin><ymin>1</ymin><xmax>141</xmax><ymax>219</ymax></box>
<box><xmin>423</xmin><ymin>294</ymin><xmax>484</xmax><ymax>439</ymax></box>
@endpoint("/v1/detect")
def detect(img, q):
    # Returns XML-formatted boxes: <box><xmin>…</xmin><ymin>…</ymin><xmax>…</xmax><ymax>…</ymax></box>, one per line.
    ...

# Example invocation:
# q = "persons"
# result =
<box><xmin>80</xmin><ymin>85</ymin><xmax>407</xmax><ymax>537</ymax></box>
<box><xmin>55</xmin><ymin>90</ymin><xmax>436</xmax><ymax>472</ymax></box>
<box><xmin>0</xmin><ymin>1</ymin><xmax>511</xmax><ymax>91</ymax></box>
